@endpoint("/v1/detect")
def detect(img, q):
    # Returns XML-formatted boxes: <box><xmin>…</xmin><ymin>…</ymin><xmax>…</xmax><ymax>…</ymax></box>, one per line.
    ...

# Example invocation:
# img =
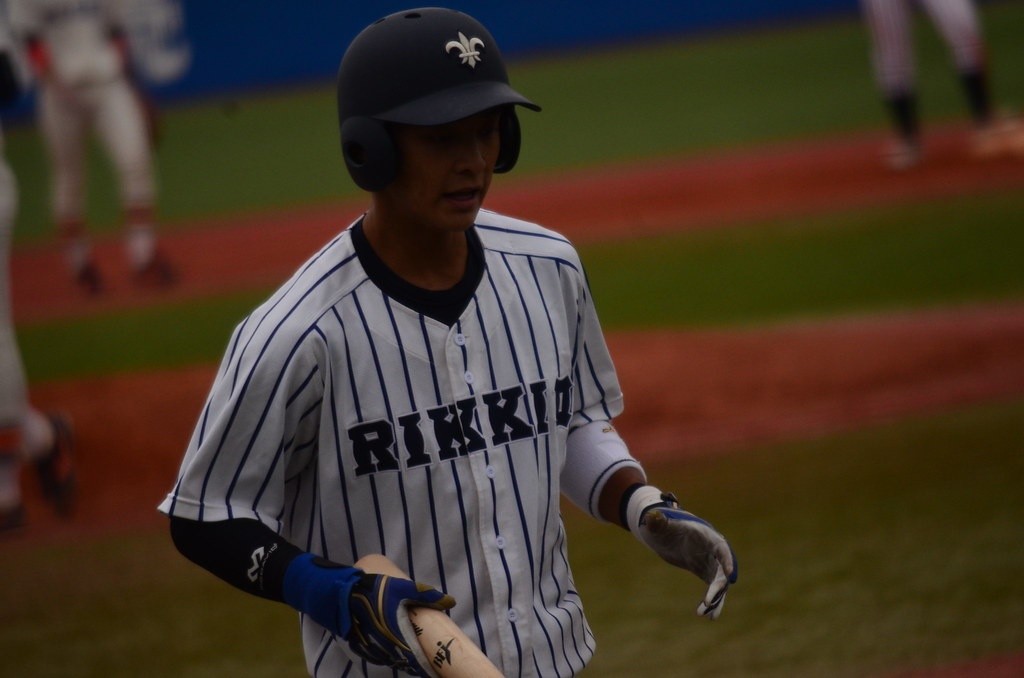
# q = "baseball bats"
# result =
<box><xmin>352</xmin><ymin>554</ymin><xmax>505</xmax><ymax>677</ymax></box>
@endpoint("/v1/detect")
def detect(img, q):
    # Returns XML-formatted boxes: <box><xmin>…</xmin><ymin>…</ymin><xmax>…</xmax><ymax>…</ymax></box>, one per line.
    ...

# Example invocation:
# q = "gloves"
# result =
<box><xmin>638</xmin><ymin>501</ymin><xmax>738</xmax><ymax>622</ymax></box>
<box><xmin>340</xmin><ymin>573</ymin><xmax>459</xmax><ymax>678</ymax></box>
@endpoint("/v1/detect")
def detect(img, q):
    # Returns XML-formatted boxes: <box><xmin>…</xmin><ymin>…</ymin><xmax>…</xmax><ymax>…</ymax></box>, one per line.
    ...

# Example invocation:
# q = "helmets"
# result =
<box><xmin>335</xmin><ymin>7</ymin><xmax>543</xmax><ymax>193</ymax></box>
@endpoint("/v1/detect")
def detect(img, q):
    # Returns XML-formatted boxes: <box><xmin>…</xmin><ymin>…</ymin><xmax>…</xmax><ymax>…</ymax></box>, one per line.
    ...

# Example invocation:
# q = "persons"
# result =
<box><xmin>0</xmin><ymin>0</ymin><xmax>189</xmax><ymax>297</ymax></box>
<box><xmin>0</xmin><ymin>162</ymin><xmax>76</xmax><ymax>529</ymax></box>
<box><xmin>155</xmin><ymin>8</ymin><xmax>741</xmax><ymax>678</ymax></box>
<box><xmin>866</xmin><ymin>0</ymin><xmax>995</xmax><ymax>168</ymax></box>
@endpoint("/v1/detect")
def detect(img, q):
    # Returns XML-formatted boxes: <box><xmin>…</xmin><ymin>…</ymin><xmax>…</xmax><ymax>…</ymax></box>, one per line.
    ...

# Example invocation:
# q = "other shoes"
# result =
<box><xmin>76</xmin><ymin>265</ymin><xmax>105</xmax><ymax>294</ymax></box>
<box><xmin>0</xmin><ymin>499</ymin><xmax>33</xmax><ymax>531</ymax></box>
<box><xmin>123</xmin><ymin>260</ymin><xmax>186</xmax><ymax>293</ymax></box>
<box><xmin>40</xmin><ymin>410</ymin><xmax>83</xmax><ymax>529</ymax></box>
